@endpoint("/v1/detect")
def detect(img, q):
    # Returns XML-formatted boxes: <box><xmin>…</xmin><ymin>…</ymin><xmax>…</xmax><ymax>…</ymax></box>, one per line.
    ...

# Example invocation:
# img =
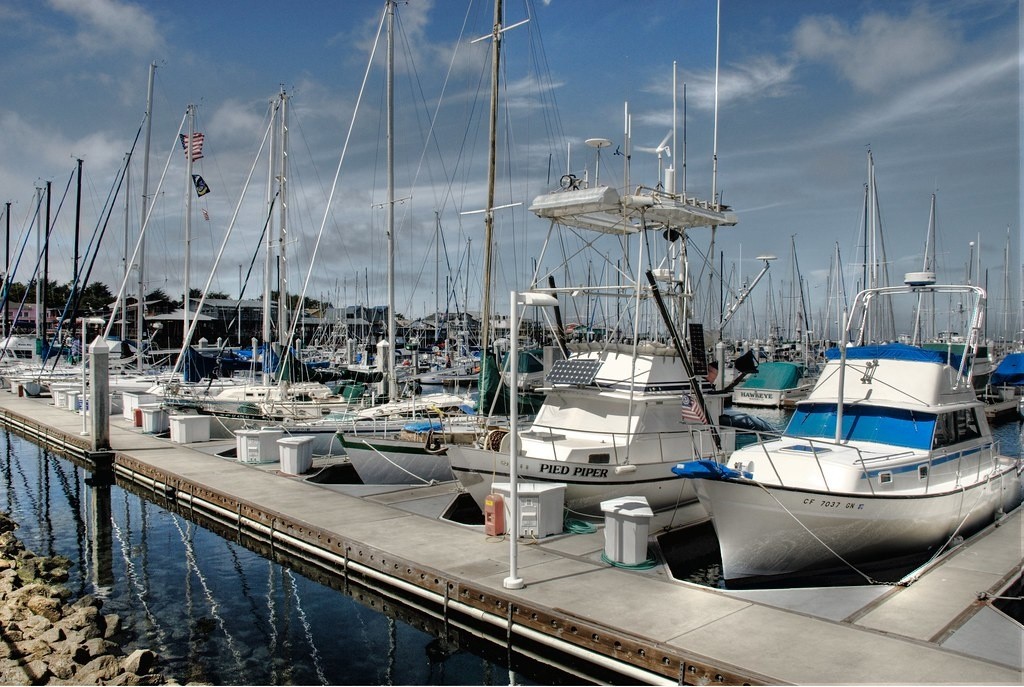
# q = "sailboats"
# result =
<box><xmin>0</xmin><ymin>0</ymin><xmax>1024</xmax><ymax>525</ymax></box>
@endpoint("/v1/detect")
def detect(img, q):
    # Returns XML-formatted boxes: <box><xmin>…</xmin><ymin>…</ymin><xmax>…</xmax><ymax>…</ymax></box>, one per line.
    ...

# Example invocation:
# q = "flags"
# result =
<box><xmin>202</xmin><ymin>209</ymin><xmax>210</xmax><ymax>221</ymax></box>
<box><xmin>681</xmin><ymin>392</ymin><xmax>707</xmax><ymax>425</ymax></box>
<box><xmin>192</xmin><ymin>174</ymin><xmax>210</xmax><ymax>197</ymax></box>
<box><xmin>179</xmin><ymin>133</ymin><xmax>204</xmax><ymax>162</ymax></box>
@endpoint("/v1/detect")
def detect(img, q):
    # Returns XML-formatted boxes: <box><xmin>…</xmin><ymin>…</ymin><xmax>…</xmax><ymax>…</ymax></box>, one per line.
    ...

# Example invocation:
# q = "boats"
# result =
<box><xmin>669</xmin><ymin>273</ymin><xmax>1022</xmax><ymax>581</ymax></box>
